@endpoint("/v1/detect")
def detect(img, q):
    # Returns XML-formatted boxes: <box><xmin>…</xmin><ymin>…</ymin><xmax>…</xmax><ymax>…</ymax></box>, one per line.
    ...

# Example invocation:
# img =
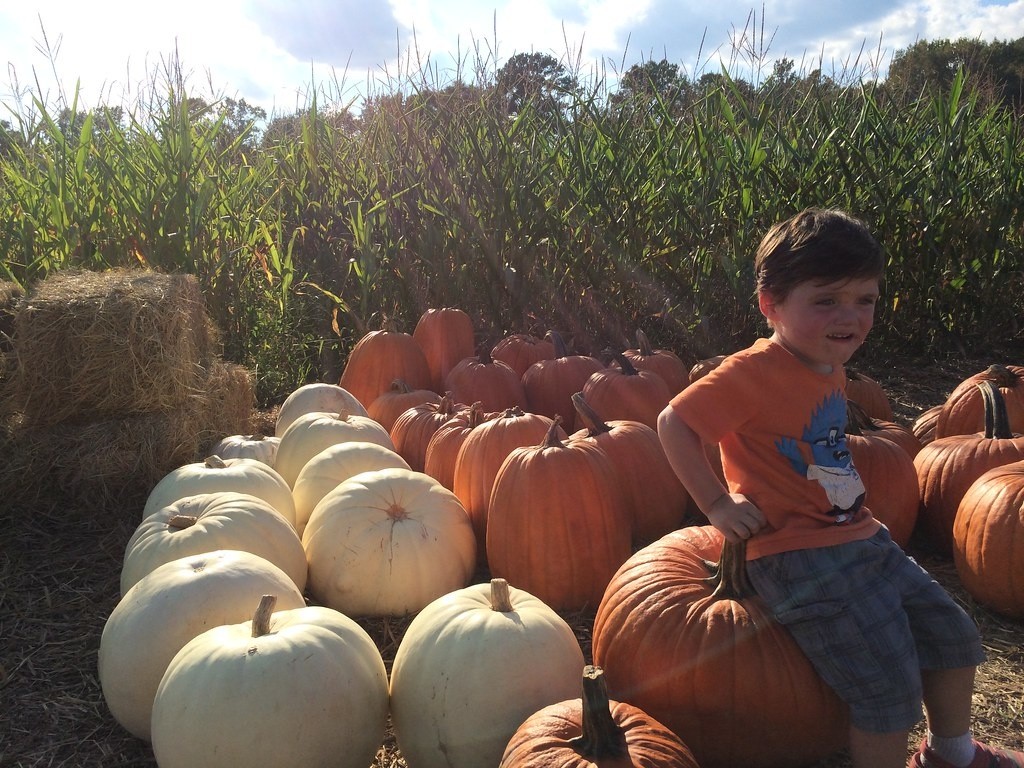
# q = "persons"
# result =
<box><xmin>656</xmin><ymin>206</ymin><xmax>1024</xmax><ymax>768</ymax></box>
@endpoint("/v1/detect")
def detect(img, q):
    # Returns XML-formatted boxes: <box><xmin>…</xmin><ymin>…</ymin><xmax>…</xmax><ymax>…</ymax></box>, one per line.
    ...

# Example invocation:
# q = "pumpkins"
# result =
<box><xmin>99</xmin><ymin>304</ymin><xmax>1024</xmax><ymax>768</ymax></box>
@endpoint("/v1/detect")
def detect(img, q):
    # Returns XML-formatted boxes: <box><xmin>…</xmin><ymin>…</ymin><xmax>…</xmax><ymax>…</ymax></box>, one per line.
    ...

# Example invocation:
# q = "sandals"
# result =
<box><xmin>910</xmin><ymin>737</ymin><xmax>1024</xmax><ymax>768</ymax></box>
